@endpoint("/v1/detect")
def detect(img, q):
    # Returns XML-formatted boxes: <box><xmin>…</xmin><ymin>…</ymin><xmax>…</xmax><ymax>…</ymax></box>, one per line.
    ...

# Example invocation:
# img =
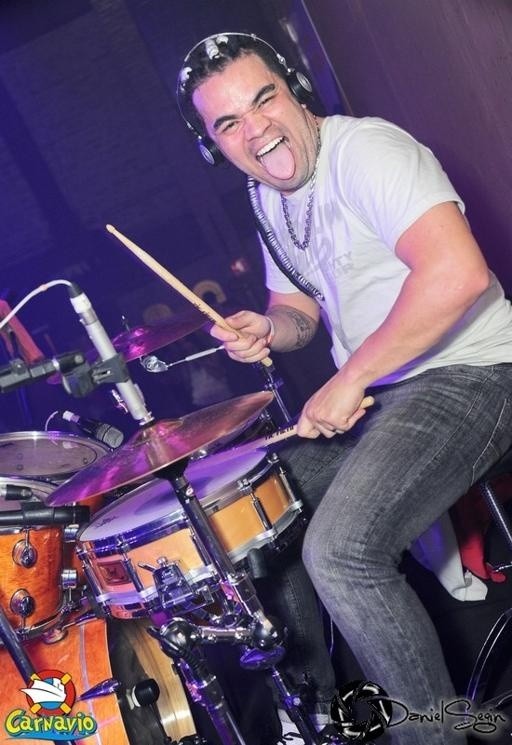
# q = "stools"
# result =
<box><xmin>464</xmin><ymin>447</ymin><xmax>511</xmax><ymax>718</ymax></box>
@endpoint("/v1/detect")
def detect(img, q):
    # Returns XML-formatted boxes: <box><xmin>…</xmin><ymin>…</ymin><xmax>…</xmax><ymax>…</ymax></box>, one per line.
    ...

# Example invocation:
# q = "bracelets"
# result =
<box><xmin>266</xmin><ymin>315</ymin><xmax>274</xmax><ymax>349</ymax></box>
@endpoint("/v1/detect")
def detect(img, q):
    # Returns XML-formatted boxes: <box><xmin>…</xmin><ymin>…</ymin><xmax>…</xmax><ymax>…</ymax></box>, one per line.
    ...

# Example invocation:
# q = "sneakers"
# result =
<box><xmin>276</xmin><ymin>726</ymin><xmax>343</xmax><ymax>744</ymax></box>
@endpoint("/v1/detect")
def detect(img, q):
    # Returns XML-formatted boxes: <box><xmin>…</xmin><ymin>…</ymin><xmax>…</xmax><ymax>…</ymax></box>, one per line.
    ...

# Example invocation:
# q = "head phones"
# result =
<box><xmin>175</xmin><ymin>31</ymin><xmax>314</xmax><ymax>167</ymax></box>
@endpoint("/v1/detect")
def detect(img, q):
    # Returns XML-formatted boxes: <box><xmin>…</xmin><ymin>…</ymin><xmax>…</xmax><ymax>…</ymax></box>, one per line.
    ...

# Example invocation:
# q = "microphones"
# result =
<box><xmin>67</xmin><ymin>282</ymin><xmax>149</xmax><ymax>421</ymax></box>
<box><xmin>57</xmin><ymin>410</ymin><xmax>124</xmax><ymax>449</ymax></box>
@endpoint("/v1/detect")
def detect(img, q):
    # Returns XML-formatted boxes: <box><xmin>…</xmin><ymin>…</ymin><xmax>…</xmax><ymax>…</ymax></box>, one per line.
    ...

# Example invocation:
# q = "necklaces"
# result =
<box><xmin>278</xmin><ymin>114</ymin><xmax>321</xmax><ymax>250</ymax></box>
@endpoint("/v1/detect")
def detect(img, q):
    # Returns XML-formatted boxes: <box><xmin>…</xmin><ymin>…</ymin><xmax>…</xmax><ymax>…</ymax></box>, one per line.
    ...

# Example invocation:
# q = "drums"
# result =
<box><xmin>1</xmin><ymin>478</ymin><xmax>81</xmax><ymax>651</ymax></box>
<box><xmin>1</xmin><ymin>432</ymin><xmax>109</xmax><ymax>487</ymax></box>
<box><xmin>76</xmin><ymin>447</ymin><xmax>306</xmax><ymax>619</ymax></box>
<box><xmin>119</xmin><ymin>618</ymin><xmax>200</xmax><ymax>743</ymax></box>
<box><xmin>1</xmin><ymin>597</ymin><xmax>198</xmax><ymax>745</ymax></box>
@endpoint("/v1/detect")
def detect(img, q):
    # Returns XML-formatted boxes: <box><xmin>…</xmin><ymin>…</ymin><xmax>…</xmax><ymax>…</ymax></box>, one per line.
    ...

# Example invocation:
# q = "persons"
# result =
<box><xmin>174</xmin><ymin>29</ymin><xmax>512</xmax><ymax>744</ymax></box>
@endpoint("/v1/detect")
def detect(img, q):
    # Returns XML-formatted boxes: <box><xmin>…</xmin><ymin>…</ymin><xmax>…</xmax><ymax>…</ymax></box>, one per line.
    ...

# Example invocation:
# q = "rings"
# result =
<box><xmin>331</xmin><ymin>428</ymin><xmax>336</xmax><ymax>432</ymax></box>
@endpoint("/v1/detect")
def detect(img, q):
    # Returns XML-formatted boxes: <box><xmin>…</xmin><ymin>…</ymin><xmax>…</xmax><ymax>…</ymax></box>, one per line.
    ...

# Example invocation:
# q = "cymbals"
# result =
<box><xmin>45</xmin><ymin>390</ymin><xmax>274</xmax><ymax>506</ymax></box>
<box><xmin>48</xmin><ymin>305</ymin><xmax>218</xmax><ymax>386</ymax></box>
